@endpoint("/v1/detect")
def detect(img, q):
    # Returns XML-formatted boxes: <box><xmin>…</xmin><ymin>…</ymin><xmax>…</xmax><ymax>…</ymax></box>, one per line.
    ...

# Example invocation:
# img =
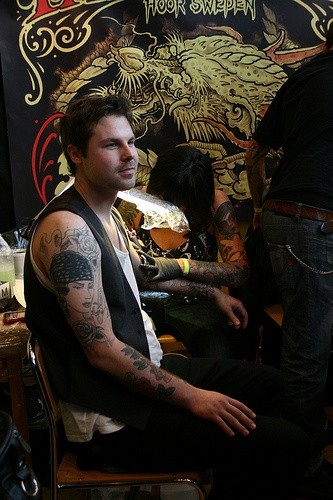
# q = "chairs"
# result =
<box><xmin>27</xmin><ymin>333</ymin><xmax>207</xmax><ymax>500</ymax></box>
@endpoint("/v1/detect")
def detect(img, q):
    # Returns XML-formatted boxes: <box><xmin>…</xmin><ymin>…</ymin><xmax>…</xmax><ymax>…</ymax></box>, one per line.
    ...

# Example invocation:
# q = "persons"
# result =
<box><xmin>117</xmin><ymin>145</ymin><xmax>252</xmax><ymax>359</ymax></box>
<box><xmin>246</xmin><ymin>21</ymin><xmax>333</xmax><ymax>489</ymax></box>
<box><xmin>23</xmin><ymin>95</ymin><xmax>317</xmax><ymax>500</ymax></box>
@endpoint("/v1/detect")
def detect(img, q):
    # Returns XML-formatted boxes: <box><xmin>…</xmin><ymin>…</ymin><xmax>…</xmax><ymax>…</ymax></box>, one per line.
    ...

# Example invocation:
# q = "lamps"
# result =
<box><xmin>33</xmin><ymin>176</ymin><xmax>191</xmax><ymax>233</ymax></box>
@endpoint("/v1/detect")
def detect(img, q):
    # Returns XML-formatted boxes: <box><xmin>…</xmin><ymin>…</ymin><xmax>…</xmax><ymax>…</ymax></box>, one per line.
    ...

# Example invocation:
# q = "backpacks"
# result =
<box><xmin>0</xmin><ymin>411</ymin><xmax>40</xmax><ymax>500</ymax></box>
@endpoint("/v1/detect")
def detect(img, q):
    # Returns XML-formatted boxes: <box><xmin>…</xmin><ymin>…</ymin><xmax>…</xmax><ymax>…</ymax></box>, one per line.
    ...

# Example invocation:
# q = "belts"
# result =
<box><xmin>262</xmin><ymin>199</ymin><xmax>333</xmax><ymax>232</ymax></box>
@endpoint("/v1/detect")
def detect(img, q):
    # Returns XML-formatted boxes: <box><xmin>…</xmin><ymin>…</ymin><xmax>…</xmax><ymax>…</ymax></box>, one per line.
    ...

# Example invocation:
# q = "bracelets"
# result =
<box><xmin>179</xmin><ymin>258</ymin><xmax>190</xmax><ymax>276</ymax></box>
<box><xmin>254</xmin><ymin>207</ymin><xmax>263</xmax><ymax>213</ymax></box>
